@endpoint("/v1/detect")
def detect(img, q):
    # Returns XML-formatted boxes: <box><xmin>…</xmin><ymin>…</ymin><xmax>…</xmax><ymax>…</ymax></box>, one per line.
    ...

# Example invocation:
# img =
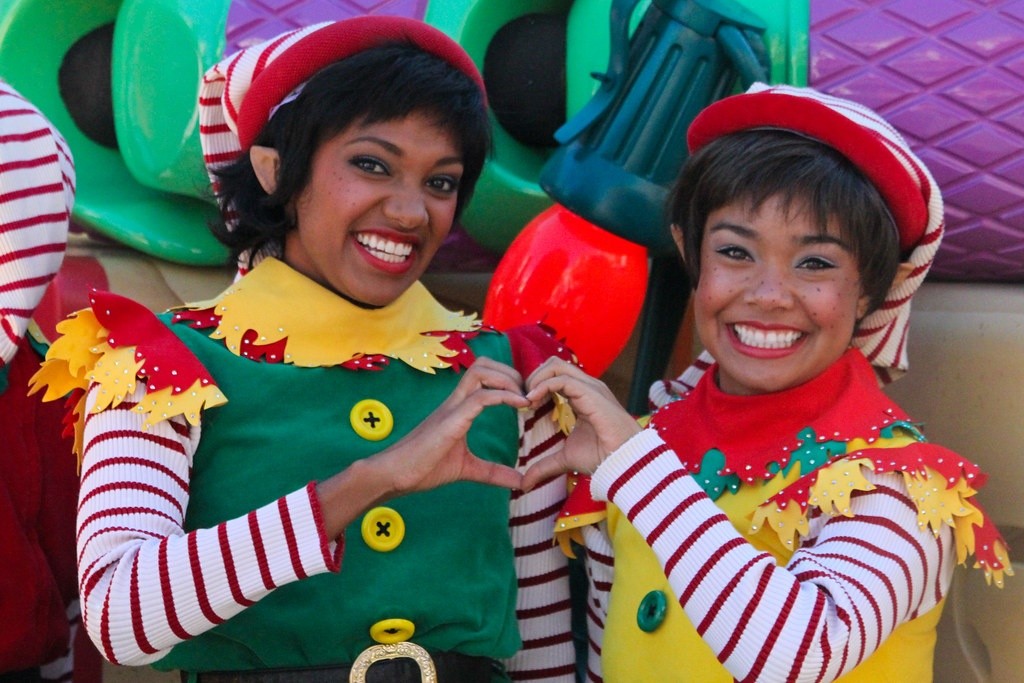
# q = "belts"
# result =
<box><xmin>179</xmin><ymin>641</ymin><xmax>507</xmax><ymax>683</ymax></box>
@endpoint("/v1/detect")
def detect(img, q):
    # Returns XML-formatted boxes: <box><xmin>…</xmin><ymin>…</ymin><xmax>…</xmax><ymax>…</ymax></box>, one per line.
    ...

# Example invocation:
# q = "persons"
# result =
<box><xmin>0</xmin><ymin>69</ymin><xmax>105</xmax><ymax>683</ymax></box>
<box><xmin>74</xmin><ymin>12</ymin><xmax>585</xmax><ymax>683</ymax></box>
<box><xmin>521</xmin><ymin>80</ymin><xmax>962</xmax><ymax>683</ymax></box>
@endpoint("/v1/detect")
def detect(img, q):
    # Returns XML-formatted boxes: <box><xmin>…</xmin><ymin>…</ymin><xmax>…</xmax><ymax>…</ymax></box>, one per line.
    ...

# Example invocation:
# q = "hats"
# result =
<box><xmin>648</xmin><ymin>81</ymin><xmax>943</xmax><ymax>415</ymax></box>
<box><xmin>0</xmin><ymin>81</ymin><xmax>76</xmax><ymax>399</ymax></box>
<box><xmin>198</xmin><ymin>14</ymin><xmax>488</xmax><ymax>288</ymax></box>
